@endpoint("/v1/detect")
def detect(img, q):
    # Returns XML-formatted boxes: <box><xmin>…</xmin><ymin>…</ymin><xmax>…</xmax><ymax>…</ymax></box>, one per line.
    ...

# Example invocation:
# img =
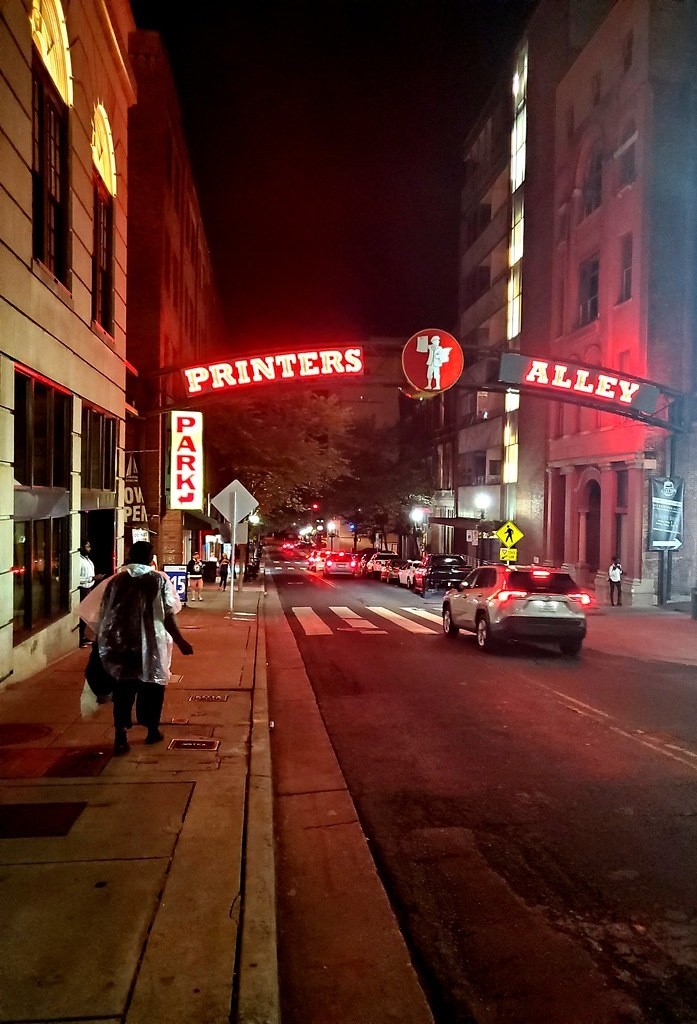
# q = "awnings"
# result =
<box><xmin>184</xmin><ymin>509</ymin><xmax>220</xmax><ymax>536</ymax></box>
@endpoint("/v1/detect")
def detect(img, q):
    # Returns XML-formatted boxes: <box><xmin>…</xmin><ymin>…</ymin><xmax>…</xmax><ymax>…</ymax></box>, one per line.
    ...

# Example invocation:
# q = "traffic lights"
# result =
<box><xmin>312</xmin><ymin>501</ymin><xmax>320</xmax><ymax>512</ymax></box>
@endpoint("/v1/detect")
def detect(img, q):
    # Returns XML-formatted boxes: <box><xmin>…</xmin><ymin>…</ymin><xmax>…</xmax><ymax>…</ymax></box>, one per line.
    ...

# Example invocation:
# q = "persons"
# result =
<box><xmin>187</xmin><ymin>551</ymin><xmax>204</xmax><ymax>601</ymax></box>
<box><xmin>608</xmin><ymin>557</ymin><xmax>623</xmax><ymax>606</ymax></box>
<box><xmin>86</xmin><ymin>540</ymin><xmax>194</xmax><ymax>756</ymax></box>
<box><xmin>218</xmin><ymin>553</ymin><xmax>231</xmax><ymax>591</ymax></box>
<box><xmin>78</xmin><ymin>538</ymin><xmax>104</xmax><ymax>647</ymax></box>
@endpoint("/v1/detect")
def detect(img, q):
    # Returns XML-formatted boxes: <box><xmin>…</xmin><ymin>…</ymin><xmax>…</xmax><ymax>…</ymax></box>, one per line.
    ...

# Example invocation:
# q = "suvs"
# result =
<box><xmin>440</xmin><ymin>562</ymin><xmax>589</xmax><ymax>657</ymax></box>
<box><xmin>411</xmin><ymin>553</ymin><xmax>474</xmax><ymax>600</ymax></box>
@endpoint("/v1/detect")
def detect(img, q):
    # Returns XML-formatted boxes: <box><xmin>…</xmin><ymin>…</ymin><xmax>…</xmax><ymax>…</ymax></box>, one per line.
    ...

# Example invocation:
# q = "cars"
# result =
<box><xmin>322</xmin><ymin>551</ymin><xmax>357</xmax><ymax>579</ymax></box>
<box><xmin>281</xmin><ymin>539</ymin><xmax>423</xmax><ymax>590</ymax></box>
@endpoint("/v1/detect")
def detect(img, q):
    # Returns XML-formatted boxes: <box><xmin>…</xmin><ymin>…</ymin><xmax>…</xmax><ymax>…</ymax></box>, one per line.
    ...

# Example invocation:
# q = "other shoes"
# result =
<box><xmin>144</xmin><ymin>731</ymin><xmax>164</xmax><ymax>745</ymax></box>
<box><xmin>198</xmin><ymin>596</ymin><xmax>203</xmax><ymax>601</ymax></box>
<box><xmin>191</xmin><ymin>597</ymin><xmax>195</xmax><ymax>601</ymax></box>
<box><xmin>114</xmin><ymin>744</ymin><xmax>130</xmax><ymax>757</ymax></box>
<box><xmin>80</xmin><ymin>638</ymin><xmax>94</xmax><ymax>649</ymax></box>
